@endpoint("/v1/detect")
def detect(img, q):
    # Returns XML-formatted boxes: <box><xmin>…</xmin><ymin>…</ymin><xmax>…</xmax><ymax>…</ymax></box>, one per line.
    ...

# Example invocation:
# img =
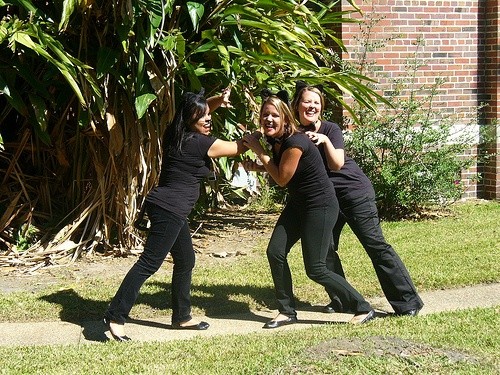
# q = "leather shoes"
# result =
<box><xmin>394</xmin><ymin>308</ymin><xmax>419</xmax><ymax>317</ymax></box>
<box><xmin>323</xmin><ymin>302</ymin><xmax>337</xmax><ymax>313</ymax></box>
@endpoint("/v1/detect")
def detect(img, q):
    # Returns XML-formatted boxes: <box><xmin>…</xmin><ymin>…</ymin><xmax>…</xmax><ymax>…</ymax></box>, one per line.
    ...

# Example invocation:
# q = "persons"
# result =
<box><xmin>240</xmin><ymin>96</ymin><xmax>374</xmax><ymax>327</ymax></box>
<box><xmin>104</xmin><ymin>84</ymin><xmax>265</xmax><ymax>342</ymax></box>
<box><xmin>289</xmin><ymin>87</ymin><xmax>424</xmax><ymax>318</ymax></box>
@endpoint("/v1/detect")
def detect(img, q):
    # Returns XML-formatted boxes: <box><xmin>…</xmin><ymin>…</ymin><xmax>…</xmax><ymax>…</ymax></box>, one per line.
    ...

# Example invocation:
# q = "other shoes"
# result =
<box><xmin>264</xmin><ymin>317</ymin><xmax>298</xmax><ymax>328</ymax></box>
<box><xmin>172</xmin><ymin>320</ymin><xmax>210</xmax><ymax>330</ymax></box>
<box><xmin>103</xmin><ymin>316</ymin><xmax>131</xmax><ymax>342</ymax></box>
<box><xmin>361</xmin><ymin>310</ymin><xmax>377</xmax><ymax>324</ymax></box>
